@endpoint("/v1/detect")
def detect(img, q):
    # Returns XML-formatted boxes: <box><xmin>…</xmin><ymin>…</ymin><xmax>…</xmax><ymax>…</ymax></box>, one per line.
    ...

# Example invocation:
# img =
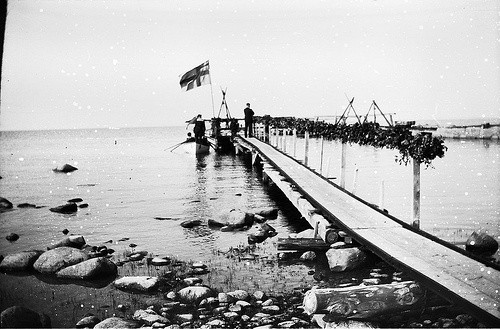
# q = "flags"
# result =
<box><xmin>179</xmin><ymin>59</ymin><xmax>211</xmax><ymax>92</ymax></box>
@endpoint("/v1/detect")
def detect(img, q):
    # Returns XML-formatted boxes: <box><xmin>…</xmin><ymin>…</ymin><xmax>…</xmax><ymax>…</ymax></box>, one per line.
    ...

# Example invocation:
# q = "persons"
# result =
<box><xmin>193</xmin><ymin>114</ymin><xmax>206</xmax><ymax>148</ymax></box>
<box><xmin>243</xmin><ymin>103</ymin><xmax>255</xmax><ymax>138</ymax></box>
